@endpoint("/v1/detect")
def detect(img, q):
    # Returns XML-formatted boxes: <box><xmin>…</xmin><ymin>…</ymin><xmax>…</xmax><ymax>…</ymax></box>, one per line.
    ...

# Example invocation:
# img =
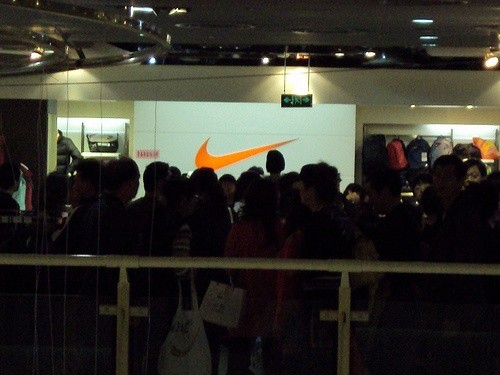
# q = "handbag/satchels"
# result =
<box><xmin>158</xmin><ymin>268</ymin><xmax>212</xmax><ymax>375</ymax></box>
<box><xmin>200</xmin><ymin>274</ymin><xmax>245</xmax><ymax>328</ymax></box>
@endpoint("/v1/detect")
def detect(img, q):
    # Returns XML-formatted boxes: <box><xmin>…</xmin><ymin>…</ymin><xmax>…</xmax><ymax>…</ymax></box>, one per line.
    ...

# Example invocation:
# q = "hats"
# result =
<box><xmin>300</xmin><ymin>163</ymin><xmax>340</xmax><ymax>205</ymax></box>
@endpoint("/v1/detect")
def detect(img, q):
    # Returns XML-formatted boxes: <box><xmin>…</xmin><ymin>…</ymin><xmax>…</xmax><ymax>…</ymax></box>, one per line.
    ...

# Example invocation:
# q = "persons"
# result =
<box><xmin>55</xmin><ymin>131</ymin><xmax>83</xmax><ymax>176</ymax></box>
<box><xmin>0</xmin><ymin>151</ymin><xmax>500</xmax><ymax>375</ymax></box>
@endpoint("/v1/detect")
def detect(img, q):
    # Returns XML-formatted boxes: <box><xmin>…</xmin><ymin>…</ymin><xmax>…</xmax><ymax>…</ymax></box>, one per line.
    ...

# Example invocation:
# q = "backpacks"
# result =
<box><xmin>454</xmin><ymin>137</ymin><xmax>500</xmax><ymax>160</ymax></box>
<box><xmin>361</xmin><ymin>134</ymin><xmax>452</xmax><ymax>172</ymax></box>
